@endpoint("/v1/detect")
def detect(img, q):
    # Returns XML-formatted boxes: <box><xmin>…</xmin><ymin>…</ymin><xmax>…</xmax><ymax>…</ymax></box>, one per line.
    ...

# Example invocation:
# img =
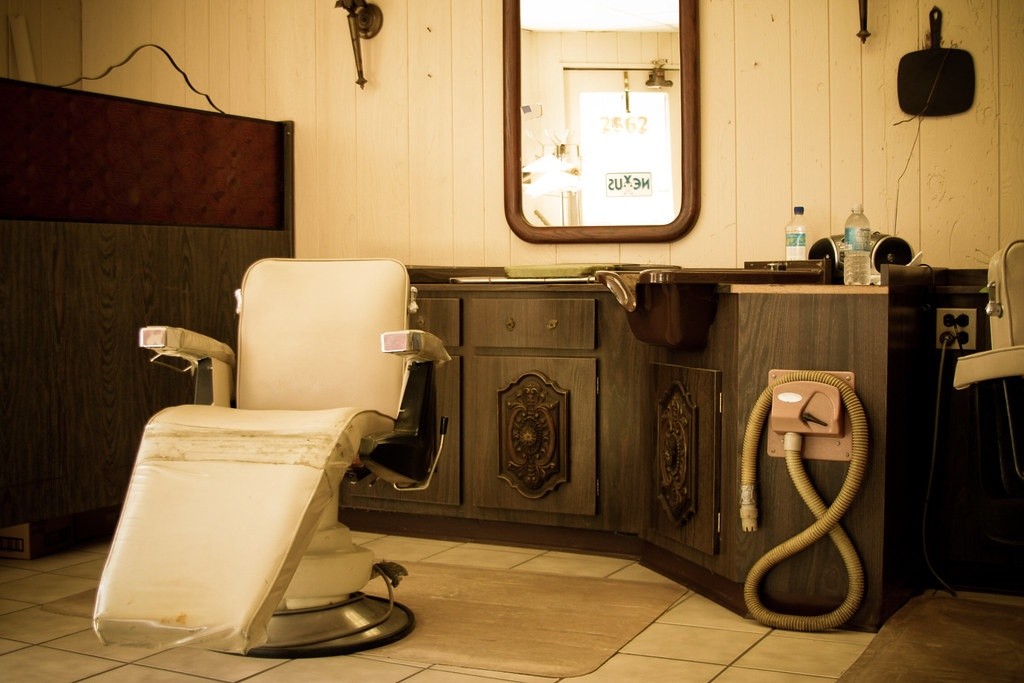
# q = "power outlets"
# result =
<box><xmin>935</xmin><ymin>308</ymin><xmax>978</xmax><ymax>351</ymax></box>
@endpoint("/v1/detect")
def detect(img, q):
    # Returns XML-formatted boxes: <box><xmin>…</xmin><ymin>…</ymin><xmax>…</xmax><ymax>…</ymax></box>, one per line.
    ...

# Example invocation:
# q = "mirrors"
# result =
<box><xmin>502</xmin><ymin>0</ymin><xmax>699</xmax><ymax>243</ymax></box>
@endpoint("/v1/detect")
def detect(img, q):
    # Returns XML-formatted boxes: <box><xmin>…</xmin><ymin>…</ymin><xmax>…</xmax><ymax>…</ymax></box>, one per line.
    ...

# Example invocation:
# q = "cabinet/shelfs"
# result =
<box><xmin>337</xmin><ymin>276</ymin><xmax>993</xmax><ymax>631</ymax></box>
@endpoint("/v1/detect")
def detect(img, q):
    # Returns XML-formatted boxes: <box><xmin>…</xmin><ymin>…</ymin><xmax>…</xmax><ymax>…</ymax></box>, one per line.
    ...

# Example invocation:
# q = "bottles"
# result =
<box><xmin>843</xmin><ymin>204</ymin><xmax>871</xmax><ymax>285</ymax></box>
<box><xmin>784</xmin><ymin>206</ymin><xmax>809</xmax><ymax>260</ymax></box>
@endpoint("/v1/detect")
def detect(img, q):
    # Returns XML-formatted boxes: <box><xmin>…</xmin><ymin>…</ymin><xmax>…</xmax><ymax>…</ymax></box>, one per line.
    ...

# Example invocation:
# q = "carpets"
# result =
<box><xmin>362</xmin><ymin>556</ymin><xmax>690</xmax><ymax>678</ymax></box>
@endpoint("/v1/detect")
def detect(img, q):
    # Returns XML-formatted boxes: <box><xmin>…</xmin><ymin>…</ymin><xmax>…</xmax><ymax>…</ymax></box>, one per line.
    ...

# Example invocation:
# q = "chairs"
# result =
<box><xmin>92</xmin><ymin>257</ymin><xmax>451</xmax><ymax>660</ymax></box>
